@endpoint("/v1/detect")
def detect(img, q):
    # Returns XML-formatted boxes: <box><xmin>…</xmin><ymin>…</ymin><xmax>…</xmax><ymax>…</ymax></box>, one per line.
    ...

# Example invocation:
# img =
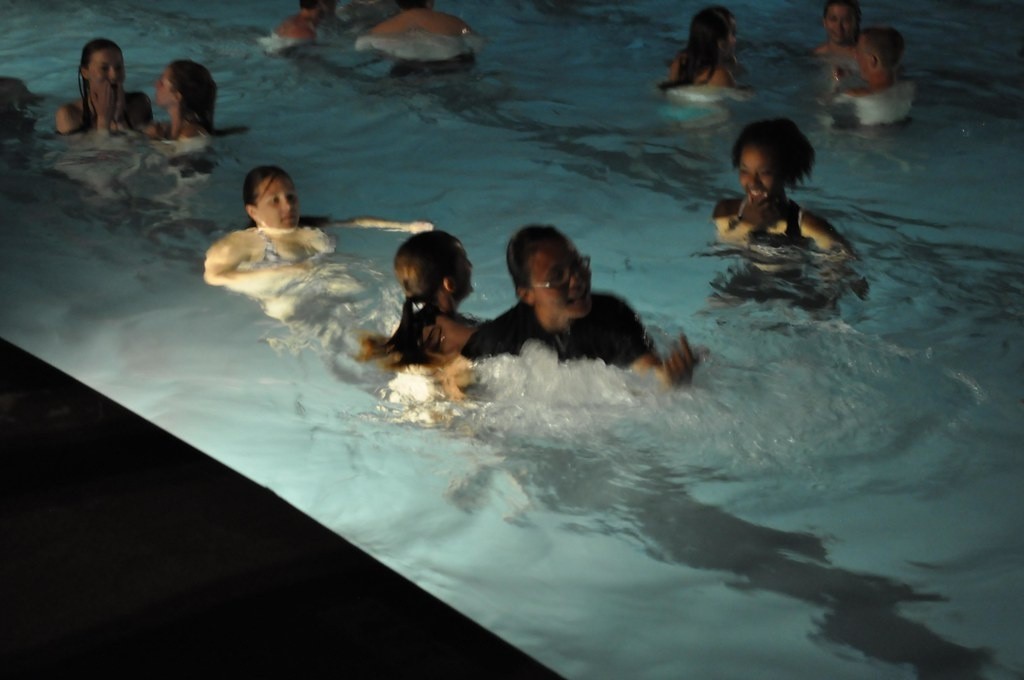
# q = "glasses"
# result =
<box><xmin>525</xmin><ymin>255</ymin><xmax>591</xmax><ymax>288</ymax></box>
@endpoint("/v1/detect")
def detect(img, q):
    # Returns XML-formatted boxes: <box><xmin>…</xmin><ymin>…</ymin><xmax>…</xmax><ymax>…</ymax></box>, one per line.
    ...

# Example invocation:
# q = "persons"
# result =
<box><xmin>390</xmin><ymin>230</ymin><xmax>481</xmax><ymax>352</ymax></box>
<box><xmin>243</xmin><ymin>166</ymin><xmax>299</xmax><ymax>229</ymax></box>
<box><xmin>154</xmin><ymin>61</ymin><xmax>217</xmax><ymax>137</ymax></box>
<box><xmin>847</xmin><ymin>27</ymin><xmax>905</xmax><ymax>97</ymax></box>
<box><xmin>275</xmin><ymin>0</ymin><xmax>338</xmax><ymax>40</ymax></box>
<box><xmin>56</xmin><ymin>39</ymin><xmax>153</xmax><ymax>134</ymax></box>
<box><xmin>817</xmin><ymin>0</ymin><xmax>861</xmax><ymax>55</ymax></box>
<box><xmin>451</xmin><ymin>223</ymin><xmax>697</xmax><ymax>387</ymax></box>
<box><xmin>372</xmin><ymin>0</ymin><xmax>467</xmax><ymax>35</ymax></box>
<box><xmin>671</xmin><ymin>6</ymin><xmax>736</xmax><ymax>86</ymax></box>
<box><xmin>714</xmin><ymin>118</ymin><xmax>838</xmax><ymax>248</ymax></box>
